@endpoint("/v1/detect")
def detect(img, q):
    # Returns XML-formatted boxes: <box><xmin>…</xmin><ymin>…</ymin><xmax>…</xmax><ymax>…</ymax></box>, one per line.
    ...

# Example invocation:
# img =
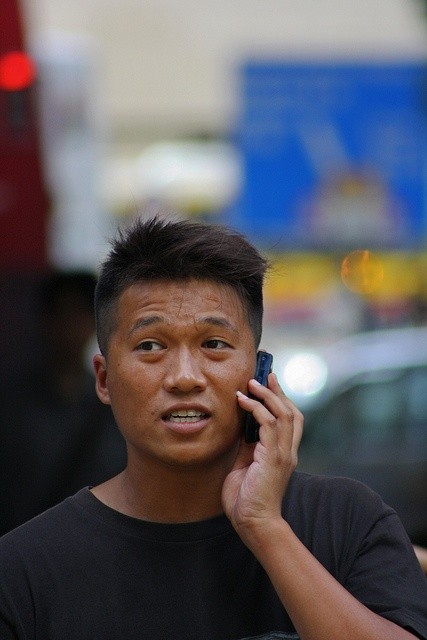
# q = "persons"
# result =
<box><xmin>1</xmin><ymin>211</ymin><xmax>426</xmax><ymax>639</ymax></box>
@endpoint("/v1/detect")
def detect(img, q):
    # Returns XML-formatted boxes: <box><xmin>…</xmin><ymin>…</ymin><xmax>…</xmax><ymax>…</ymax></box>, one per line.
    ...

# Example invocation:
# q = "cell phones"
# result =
<box><xmin>245</xmin><ymin>351</ymin><xmax>274</xmax><ymax>442</ymax></box>
